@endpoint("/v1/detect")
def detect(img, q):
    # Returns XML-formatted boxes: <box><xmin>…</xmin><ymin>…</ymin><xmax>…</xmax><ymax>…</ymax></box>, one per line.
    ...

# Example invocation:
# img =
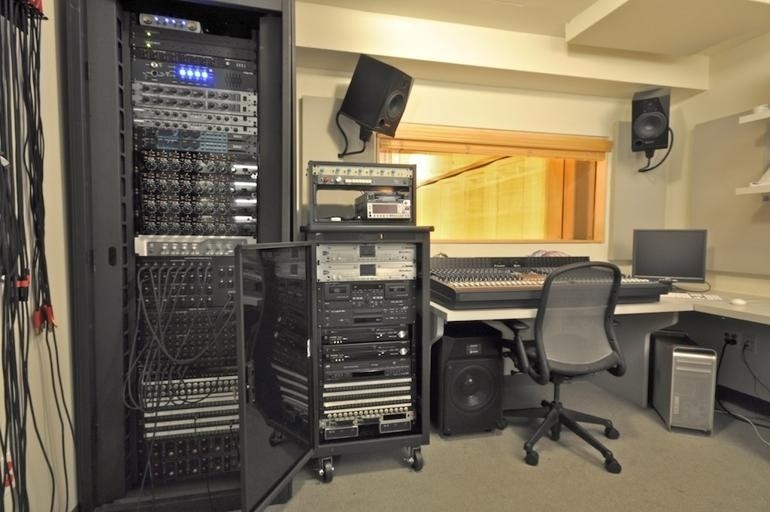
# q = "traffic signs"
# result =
<box><xmin>722</xmin><ymin>330</ymin><xmax>757</xmax><ymax>352</ymax></box>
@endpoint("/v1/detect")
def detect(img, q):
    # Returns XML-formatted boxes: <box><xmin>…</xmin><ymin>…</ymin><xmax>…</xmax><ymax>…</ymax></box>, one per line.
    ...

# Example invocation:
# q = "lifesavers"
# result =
<box><xmin>650</xmin><ymin>329</ymin><xmax>718</xmax><ymax>436</ymax></box>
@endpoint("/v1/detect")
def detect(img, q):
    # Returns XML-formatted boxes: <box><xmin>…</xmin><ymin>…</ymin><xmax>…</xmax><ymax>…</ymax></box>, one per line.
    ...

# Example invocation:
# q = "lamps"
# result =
<box><xmin>493</xmin><ymin>253</ymin><xmax>627</xmax><ymax>474</ymax></box>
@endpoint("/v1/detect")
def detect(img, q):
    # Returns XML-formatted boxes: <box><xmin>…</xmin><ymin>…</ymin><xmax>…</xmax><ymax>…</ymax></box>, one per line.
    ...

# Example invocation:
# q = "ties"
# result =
<box><xmin>631</xmin><ymin>228</ymin><xmax>708</xmax><ymax>294</ymax></box>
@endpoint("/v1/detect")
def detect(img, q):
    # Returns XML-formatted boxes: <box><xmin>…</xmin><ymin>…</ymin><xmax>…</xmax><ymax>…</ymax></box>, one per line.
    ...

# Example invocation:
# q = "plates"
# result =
<box><xmin>736</xmin><ymin>105</ymin><xmax>769</xmax><ymax>196</ymax></box>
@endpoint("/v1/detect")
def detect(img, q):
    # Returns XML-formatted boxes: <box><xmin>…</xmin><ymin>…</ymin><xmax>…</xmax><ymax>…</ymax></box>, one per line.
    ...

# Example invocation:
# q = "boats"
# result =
<box><xmin>340</xmin><ymin>53</ymin><xmax>413</xmax><ymax>143</ymax></box>
<box><xmin>431</xmin><ymin>320</ymin><xmax>504</xmax><ymax>439</ymax></box>
<box><xmin>631</xmin><ymin>87</ymin><xmax>671</xmax><ymax>159</ymax></box>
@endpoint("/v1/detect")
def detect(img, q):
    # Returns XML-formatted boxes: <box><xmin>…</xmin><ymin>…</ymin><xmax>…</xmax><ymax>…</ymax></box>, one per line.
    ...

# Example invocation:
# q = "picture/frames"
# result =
<box><xmin>428</xmin><ymin>289</ymin><xmax>770</xmax><ymax>339</ymax></box>
